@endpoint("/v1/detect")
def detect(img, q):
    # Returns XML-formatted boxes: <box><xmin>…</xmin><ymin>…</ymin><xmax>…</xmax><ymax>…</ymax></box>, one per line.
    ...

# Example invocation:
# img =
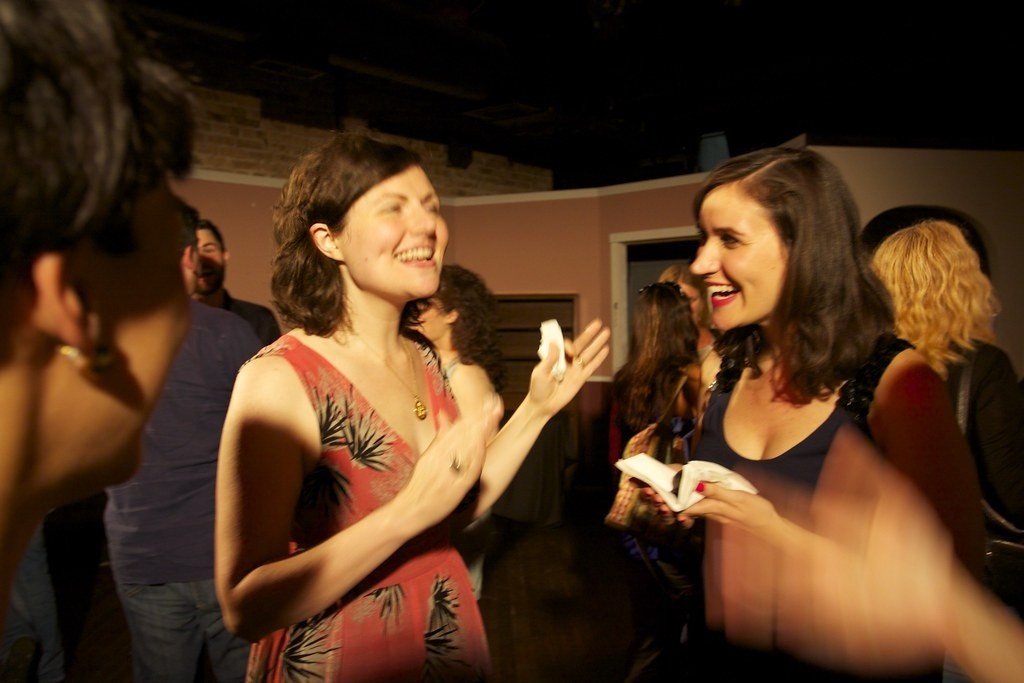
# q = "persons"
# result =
<box><xmin>0</xmin><ymin>0</ymin><xmax>192</xmax><ymax>620</ymax></box>
<box><xmin>215</xmin><ymin>134</ymin><xmax>611</xmax><ymax>683</ymax></box>
<box><xmin>103</xmin><ymin>199</ymin><xmax>284</xmax><ymax>683</ymax></box>
<box><xmin>614</xmin><ymin>146</ymin><xmax>1024</xmax><ymax>683</ymax></box>
<box><xmin>403</xmin><ymin>263</ymin><xmax>504</xmax><ymax>602</ymax></box>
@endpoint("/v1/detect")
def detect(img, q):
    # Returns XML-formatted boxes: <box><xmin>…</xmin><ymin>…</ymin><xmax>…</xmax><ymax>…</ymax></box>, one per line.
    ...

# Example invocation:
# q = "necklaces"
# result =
<box><xmin>345</xmin><ymin>322</ymin><xmax>427</xmax><ymax>420</ymax></box>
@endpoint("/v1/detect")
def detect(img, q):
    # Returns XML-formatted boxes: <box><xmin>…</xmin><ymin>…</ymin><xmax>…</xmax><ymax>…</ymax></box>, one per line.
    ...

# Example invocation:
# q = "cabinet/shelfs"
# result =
<box><xmin>490</xmin><ymin>292</ymin><xmax>578</xmax><ymax>462</ymax></box>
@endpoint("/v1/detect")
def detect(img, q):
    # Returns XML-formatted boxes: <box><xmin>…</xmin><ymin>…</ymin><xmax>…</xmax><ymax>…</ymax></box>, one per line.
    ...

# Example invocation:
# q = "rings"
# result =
<box><xmin>450</xmin><ymin>459</ymin><xmax>463</xmax><ymax>472</ymax></box>
